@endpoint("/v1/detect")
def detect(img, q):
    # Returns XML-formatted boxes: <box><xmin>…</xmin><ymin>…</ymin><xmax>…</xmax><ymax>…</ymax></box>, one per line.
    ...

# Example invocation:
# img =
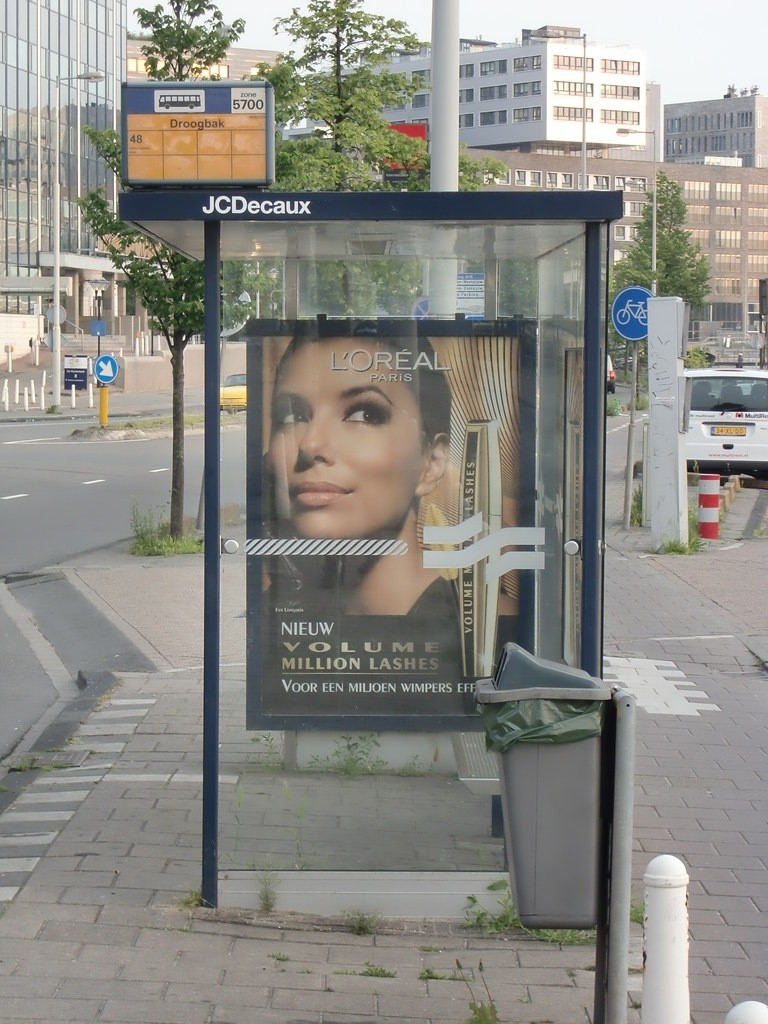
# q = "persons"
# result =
<box><xmin>262</xmin><ymin>335</ymin><xmax>457</xmax><ymax>617</ymax></box>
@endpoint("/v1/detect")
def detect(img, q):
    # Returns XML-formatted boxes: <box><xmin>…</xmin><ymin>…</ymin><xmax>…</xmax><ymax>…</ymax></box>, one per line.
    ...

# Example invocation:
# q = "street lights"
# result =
<box><xmin>267</xmin><ymin>267</ymin><xmax>281</xmax><ymax>320</ymax></box>
<box><xmin>46</xmin><ymin>72</ymin><xmax>106</xmax><ymax>417</ymax></box>
<box><xmin>615</xmin><ymin>127</ymin><xmax>659</xmax><ymax>296</ymax></box>
<box><xmin>528</xmin><ymin>28</ymin><xmax>589</xmax><ymax>192</ymax></box>
<box><xmin>88</xmin><ymin>276</ymin><xmax>110</xmax><ymax>388</ymax></box>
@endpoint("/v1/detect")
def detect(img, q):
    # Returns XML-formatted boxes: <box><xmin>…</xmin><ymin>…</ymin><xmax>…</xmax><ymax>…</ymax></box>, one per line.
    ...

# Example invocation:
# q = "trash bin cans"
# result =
<box><xmin>474</xmin><ymin>643</ymin><xmax>613</xmax><ymax>931</ymax></box>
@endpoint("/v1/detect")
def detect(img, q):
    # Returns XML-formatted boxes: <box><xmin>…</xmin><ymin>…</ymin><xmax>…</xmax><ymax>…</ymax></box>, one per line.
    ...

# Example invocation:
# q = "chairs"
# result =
<box><xmin>697</xmin><ymin>381</ymin><xmax>716</xmax><ymax>398</ymax></box>
<box><xmin>751</xmin><ymin>383</ymin><xmax>768</xmax><ymax>400</ymax></box>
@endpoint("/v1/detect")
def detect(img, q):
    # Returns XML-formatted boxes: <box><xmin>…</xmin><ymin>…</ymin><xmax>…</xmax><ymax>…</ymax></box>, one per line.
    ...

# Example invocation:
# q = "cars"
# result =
<box><xmin>682</xmin><ymin>366</ymin><xmax>768</xmax><ymax>480</ymax></box>
<box><xmin>219</xmin><ymin>373</ymin><xmax>246</xmax><ymax>415</ymax></box>
<box><xmin>607</xmin><ymin>355</ymin><xmax>616</xmax><ymax>395</ymax></box>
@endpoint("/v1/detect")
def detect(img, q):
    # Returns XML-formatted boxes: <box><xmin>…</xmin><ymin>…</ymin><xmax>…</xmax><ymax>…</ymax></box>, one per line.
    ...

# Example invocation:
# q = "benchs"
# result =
<box><xmin>720</xmin><ymin>385</ymin><xmax>768</xmax><ymax>408</ymax></box>
